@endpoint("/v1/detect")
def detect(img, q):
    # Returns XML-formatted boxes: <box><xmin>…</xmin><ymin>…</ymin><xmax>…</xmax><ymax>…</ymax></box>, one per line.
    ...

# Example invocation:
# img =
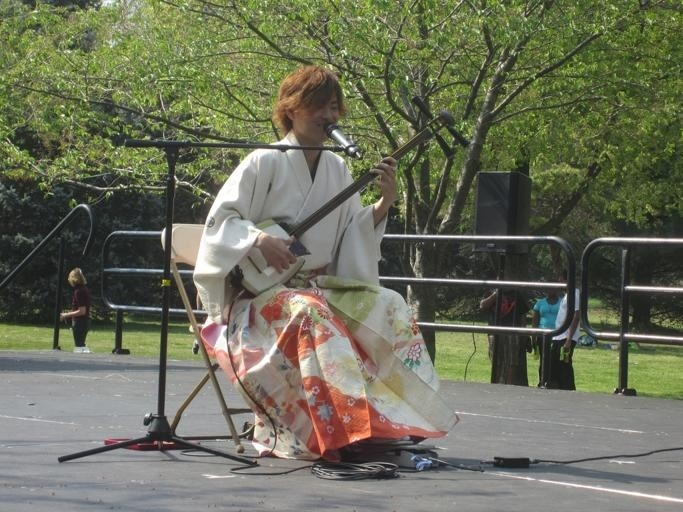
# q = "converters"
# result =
<box><xmin>493</xmin><ymin>456</ymin><xmax>530</xmax><ymax>468</ymax></box>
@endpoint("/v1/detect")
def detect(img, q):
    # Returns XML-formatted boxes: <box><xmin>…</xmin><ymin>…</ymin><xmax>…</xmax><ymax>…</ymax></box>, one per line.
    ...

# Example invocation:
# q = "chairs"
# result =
<box><xmin>159</xmin><ymin>222</ymin><xmax>255</xmax><ymax>454</ymax></box>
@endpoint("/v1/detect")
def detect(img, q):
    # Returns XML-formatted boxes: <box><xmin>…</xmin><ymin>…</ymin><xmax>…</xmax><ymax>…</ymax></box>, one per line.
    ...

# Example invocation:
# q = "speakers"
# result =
<box><xmin>470</xmin><ymin>170</ymin><xmax>532</xmax><ymax>255</ymax></box>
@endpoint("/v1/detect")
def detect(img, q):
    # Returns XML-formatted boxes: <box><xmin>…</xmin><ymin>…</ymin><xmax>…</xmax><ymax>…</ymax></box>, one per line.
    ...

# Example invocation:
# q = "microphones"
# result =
<box><xmin>323</xmin><ymin>122</ymin><xmax>364</xmax><ymax>160</ymax></box>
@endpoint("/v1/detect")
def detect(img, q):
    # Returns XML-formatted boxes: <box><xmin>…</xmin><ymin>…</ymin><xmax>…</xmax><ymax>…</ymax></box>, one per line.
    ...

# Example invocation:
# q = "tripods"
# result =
<box><xmin>58</xmin><ymin>139</ymin><xmax>343</xmax><ymax>467</ymax></box>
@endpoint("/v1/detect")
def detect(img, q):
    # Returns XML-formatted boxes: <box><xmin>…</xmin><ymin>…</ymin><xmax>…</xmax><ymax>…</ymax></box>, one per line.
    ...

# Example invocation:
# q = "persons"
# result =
<box><xmin>60</xmin><ymin>267</ymin><xmax>90</xmax><ymax>347</ymax></box>
<box><xmin>532</xmin><ymin>285</ymin><xmax>561</xmax><ymax>387</ymax></box>
<box><xmin>479</xmin><ymin>272</ymin><xmax>530</xmax><ymax>361</ymax></box>
<box><xmin>550</xmin><ymin>272</ymin><xmax>581</xmax><ymax>390</ymax></box>
<box><xmin>193</xmin><ymin>66</ymin><xmax>459</xmax><ymax>464</ymax></box>
